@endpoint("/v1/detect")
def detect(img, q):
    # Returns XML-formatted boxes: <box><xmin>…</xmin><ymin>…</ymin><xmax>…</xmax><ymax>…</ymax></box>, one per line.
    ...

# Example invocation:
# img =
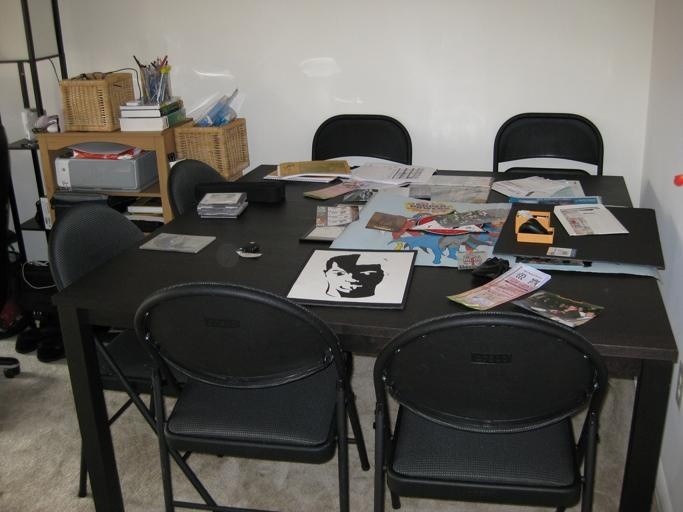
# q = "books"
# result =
<box><xmin>193</xmin><ymin>191</ymin><xmax>249</xmax><ymax>221</ymax></box>
<box><xmin>121</xmin><ymin>197</ymin><xmax>165</xmax><ymax>225</ymax></box>
<box><xmin>116</xmin><ymin>95</ymin><xmax>187</xmax><ymax>135</ymax></box>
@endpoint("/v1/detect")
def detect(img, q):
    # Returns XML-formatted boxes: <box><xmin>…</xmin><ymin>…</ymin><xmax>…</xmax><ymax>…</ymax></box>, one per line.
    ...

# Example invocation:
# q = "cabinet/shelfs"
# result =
<box><xmin>35</xmin><ymin>118</ymin><xmax>183</xmax><ymax>234</ymax></box>
<box><xmin>0</xmin><ymin>0</ymin><xmax>67</xmax><ymax>311</ymax></box>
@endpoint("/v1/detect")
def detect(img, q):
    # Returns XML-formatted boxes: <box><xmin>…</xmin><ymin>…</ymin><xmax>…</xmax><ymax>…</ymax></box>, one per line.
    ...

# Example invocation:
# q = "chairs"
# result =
<box><xmin>166</xmin><ymin>159</ymin><xmax>230</xmax><ymax>218</ymax></box>
<box><xmin>492</xmin><ymin>111</ymin><xmax>605</xmax><ymax>178</ymax></box>
<box><xmin>309</xmin><ymin>109</ymin><xmax>415</xmax><ymax>167</ymax></box>
<box><xmin>45</xmin><ymin>204</ymin><xmax>218</xmax><ymax>511</ymax></box>
<box><xmin>128</xmin><ymin>277</ymin><xmax>372</xmax><ymax>512</ymax></box>
<box><xmin>366</xmin><ymin>306</ymin><xmax>610</xmax><ymax>512</ymax></box>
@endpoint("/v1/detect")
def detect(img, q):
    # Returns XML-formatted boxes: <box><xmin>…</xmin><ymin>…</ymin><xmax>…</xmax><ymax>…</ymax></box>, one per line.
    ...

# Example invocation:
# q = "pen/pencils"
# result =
<box><xmin>133</xmin><ymin>55</ymin><xmax>170</xmax><ymax>104</ymax></box>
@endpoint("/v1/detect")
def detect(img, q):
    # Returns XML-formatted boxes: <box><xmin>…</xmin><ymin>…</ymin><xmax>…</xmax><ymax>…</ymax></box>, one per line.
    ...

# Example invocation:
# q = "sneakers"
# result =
<box><xmin>38</xmin><ymin>336</ymin><xmax>66</xmax><ymax>364</ymax></box>
<box><xmin>17</xmin><ymin>322</ymin><xmax>51</xmax><ymax>356</ymax></box>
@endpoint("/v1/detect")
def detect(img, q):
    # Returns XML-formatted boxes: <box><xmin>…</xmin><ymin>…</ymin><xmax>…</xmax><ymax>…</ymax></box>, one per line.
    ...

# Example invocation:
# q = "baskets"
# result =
<box><xmin>59</xmin><ymin>69</ymin><xmax>133</xmax><ymax>131</ymax></box>
<box><xmin>175</xmin><ymin>108</ymin><xmax>250</xmax><ymax>179</ymax></box>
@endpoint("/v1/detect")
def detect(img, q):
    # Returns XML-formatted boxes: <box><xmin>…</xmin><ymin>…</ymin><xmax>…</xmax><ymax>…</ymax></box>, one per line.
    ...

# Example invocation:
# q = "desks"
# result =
<box><xmin>48</xmin><ymin>158</ymin><xmax>679</xmax><ymax>511</ymax></box>
